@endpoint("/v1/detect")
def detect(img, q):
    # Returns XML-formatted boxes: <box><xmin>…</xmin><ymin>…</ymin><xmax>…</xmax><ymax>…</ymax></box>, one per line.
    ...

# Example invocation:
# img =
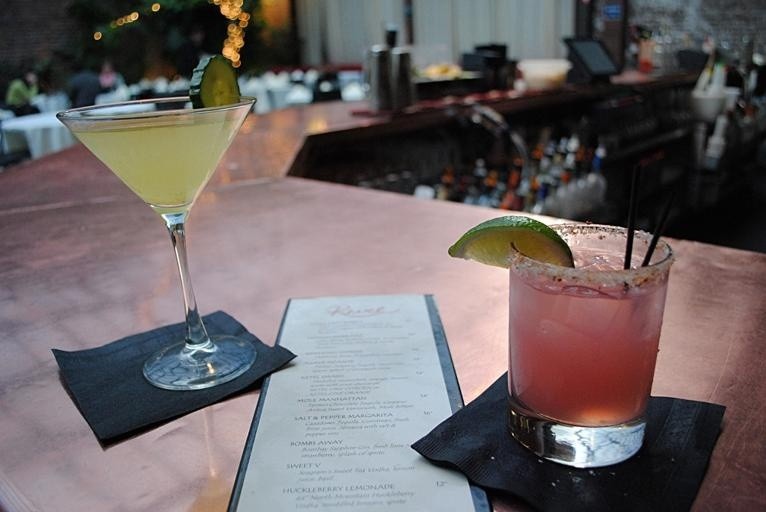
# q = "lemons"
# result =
<box><xmin>449</xmin><ymin>217</ymin><xmax>574</xmax><ymax>269</ymax></box>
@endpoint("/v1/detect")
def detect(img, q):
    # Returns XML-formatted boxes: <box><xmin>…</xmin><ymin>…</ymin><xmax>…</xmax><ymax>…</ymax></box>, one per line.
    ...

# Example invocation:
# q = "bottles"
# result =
<box><xmin>411</xmin><ymin>129</ymin><xmax>609</xmax><ymax>220</ymax></box>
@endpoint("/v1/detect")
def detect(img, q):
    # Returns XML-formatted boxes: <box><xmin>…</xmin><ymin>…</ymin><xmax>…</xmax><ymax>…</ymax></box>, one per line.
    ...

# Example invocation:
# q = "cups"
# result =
<box><xmin>507</xmin><ymin>224</ymin><xmax>676</xmax><ymax>469</ymax></box>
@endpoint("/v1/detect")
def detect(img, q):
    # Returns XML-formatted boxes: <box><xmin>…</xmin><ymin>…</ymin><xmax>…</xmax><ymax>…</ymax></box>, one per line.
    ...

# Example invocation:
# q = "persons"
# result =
<box><xmin>63</xmin><ymin>56</ymin><xmax>126</xmax><ymax>108</ymax></box>
<box><xmin>3</xmin><ymin>67</ymin><xmax>41</xmax><ymax>118</ymax></box>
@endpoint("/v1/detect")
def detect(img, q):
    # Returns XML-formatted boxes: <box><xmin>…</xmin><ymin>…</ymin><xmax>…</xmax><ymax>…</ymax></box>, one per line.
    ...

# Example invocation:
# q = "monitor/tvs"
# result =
<box><xmin>564</xmin><ymin>36</ymin><xmax>621</xmax><ymax>76</ymax></box>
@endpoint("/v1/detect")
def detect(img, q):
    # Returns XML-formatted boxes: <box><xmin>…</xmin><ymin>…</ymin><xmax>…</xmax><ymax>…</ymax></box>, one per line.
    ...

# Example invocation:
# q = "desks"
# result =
<box><xmin>1</xmin><ymin>100</ymin><xmax>157</xmax><ymax>157</ymax></box>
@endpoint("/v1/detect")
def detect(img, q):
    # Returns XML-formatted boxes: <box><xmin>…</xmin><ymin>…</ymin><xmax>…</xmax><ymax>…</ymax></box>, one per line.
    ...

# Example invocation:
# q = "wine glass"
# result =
<box><xmin>55</xmin><ymin>96</ymin><xmax>258</xmax><ymax>391</ymax></box>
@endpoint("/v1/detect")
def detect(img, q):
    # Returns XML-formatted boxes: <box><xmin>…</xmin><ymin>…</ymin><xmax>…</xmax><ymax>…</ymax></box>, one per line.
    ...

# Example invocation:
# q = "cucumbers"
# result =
<box><xmin>189</xmin><ymin>53</ymin><xmax>242</xmax><ymax>109</ymax></box>
